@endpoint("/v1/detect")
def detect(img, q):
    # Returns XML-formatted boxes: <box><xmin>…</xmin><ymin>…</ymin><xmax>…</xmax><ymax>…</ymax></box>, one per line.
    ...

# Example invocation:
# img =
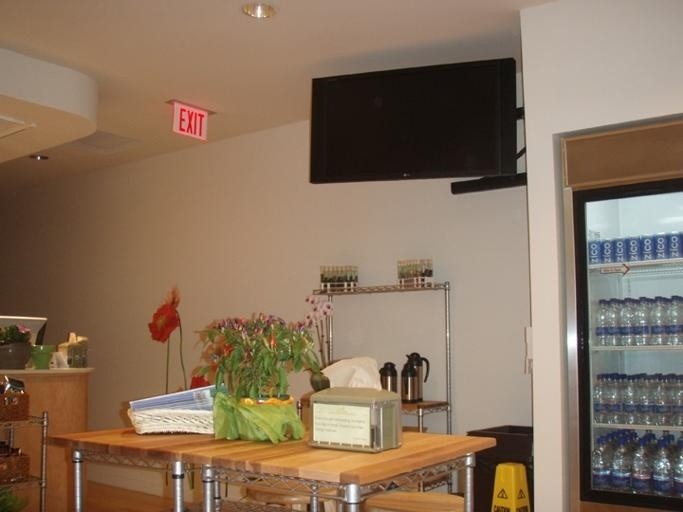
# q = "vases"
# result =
<box><xmin>232</xmin><ymin>395</ymin><xmax>294</xmax><ymax>441</ymax></box>
<box><xmin>309</xmin><ymin>364</ymin><xmax>335</xmax><ymax>392</ymax></box>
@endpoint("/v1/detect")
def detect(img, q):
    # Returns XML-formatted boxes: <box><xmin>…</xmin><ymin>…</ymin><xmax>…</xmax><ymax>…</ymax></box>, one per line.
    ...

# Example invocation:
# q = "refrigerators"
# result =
<box><xmin>572</xmin><ymin>178</ymin><xmax>683</xmax><ymax>493</ymax></box>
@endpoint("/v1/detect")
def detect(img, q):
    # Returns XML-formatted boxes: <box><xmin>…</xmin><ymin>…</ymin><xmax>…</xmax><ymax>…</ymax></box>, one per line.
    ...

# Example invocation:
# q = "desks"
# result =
<box><xmin>46</xmin><ymin>427</ymin><xmax>496</xmax><ymax>512</ymax></box>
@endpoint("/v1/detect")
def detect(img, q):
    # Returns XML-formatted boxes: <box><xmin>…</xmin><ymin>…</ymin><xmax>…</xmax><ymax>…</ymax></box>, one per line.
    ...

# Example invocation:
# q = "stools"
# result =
<box><xmin>363</xmin><ymin>492</ymin><xmax>464</xmax><ymax>512</ymax></box>
<box><xmin>246</xmin><ymin>480</ymin><xmax>340</xmax><ymax>512</ymax></box>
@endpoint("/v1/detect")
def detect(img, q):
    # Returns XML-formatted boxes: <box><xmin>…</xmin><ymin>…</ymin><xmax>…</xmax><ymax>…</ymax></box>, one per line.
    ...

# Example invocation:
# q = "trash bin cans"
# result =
<box><xmin>466</xmin><ymin>425</ymin><xmax>534</xmax><ymax>512</ymax></box>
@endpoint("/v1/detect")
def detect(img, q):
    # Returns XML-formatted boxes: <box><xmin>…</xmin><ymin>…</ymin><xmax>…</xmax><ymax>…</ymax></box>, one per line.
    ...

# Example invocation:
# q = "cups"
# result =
<box><xmin>32</xmin><ymin>343</ymin><xmax>56</xmax><ymax>369</ymax></box>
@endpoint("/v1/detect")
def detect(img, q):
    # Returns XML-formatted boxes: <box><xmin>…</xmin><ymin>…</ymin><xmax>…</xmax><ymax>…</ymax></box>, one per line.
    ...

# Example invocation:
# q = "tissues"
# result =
<box><xmin>304</xmin><ymin>356</ymin><xmax>403</xmax><ymax>452</ymax></box>
<box><xmin>58</xmin><ymin>332</ymin><xmax>87</xmax><ymax>367</ymax></box>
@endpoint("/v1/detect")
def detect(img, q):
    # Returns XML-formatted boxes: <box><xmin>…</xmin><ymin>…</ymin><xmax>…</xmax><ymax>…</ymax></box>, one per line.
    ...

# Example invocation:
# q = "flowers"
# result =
<box><xmin>305</xmin><ymin>296</ymin><xmax>334</xmax><ymax>368</ymax></box>
<box><xmin>191</xmin><ymin>312</ymin><xmax>321</xmax><ymax>404</ymax></box>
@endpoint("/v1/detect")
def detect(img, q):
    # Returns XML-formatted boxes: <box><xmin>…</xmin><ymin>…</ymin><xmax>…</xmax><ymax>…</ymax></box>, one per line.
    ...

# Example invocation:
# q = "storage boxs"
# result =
<box><xmin>308</xmin><ymin>387</ymin><xmax>400</xmax><ymax>454</ymax></box>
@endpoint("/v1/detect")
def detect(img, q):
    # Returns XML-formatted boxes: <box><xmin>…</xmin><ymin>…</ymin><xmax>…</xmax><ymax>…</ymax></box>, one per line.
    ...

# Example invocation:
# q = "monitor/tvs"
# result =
<box><xmin>309</xmin><ymin>57</ymin><xmax>517</xmax><ymax>185</ymax></box>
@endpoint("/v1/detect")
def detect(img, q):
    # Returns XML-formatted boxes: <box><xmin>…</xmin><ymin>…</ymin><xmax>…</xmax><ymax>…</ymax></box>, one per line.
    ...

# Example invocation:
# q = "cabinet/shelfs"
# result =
<box><xmin>560</xmin><ymin>120</ymin><xmax>683</xmax><ymax>512</ymax></box>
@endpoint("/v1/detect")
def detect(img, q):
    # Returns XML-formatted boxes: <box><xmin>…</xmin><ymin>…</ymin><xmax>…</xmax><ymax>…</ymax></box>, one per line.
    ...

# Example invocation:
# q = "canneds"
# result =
<box><xmin>668</xmin><ymin>231</ymin><xmax>683</xmax><ymax>258</ymax></box>
<box><xmin>614</xmin><ymin>237</ymin><xmax>626</xmax><ymax>262</ymax></box>
<box><xmin>626</xmin><ymin>235</ymin><xmax>641</xmax><ymax>262</ymax></box>
<box><xmin>655</xmin><ymin>232</ymin><xmax>669</xmax><ymax>260</ymax></box>
<box><xmin>641</xmin><ymin>234</ymin><xmax>655</xmax><ymax>260</ymax></box>
<box><xmin>588</xmin><ymin>240</ymin><xmax>600</xmax><ymax>264</ymax></box>
<box><xmin>601</xmin><ymin>239</ymin><xmax>613</xmax><ymax>264</ymax></box>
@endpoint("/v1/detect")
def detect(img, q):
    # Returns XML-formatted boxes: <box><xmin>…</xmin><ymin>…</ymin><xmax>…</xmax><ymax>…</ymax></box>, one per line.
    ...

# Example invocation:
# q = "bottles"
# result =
<box><xmin>595</xmin><ymin>295</ymin><xmax>683</xmax><ymax>346</ymax></box>
<box><xmin>591</xmin><ymin>429</ymin><xmax>683</xmax><ymax>498</ymax></box>
<box><xmin>396</xmin><ymin>258</ymin><xmax>434</xmax><ymax>288</ymax></box>
<box><xmin>594</xmin><ymin>373</ymin><xmax>683</xmax><ymax>427</ymax></box>
<box><xmin>319</xmin><ymin>264</ymin><xmax>359</xmax><ymax>292</ymax></box>
